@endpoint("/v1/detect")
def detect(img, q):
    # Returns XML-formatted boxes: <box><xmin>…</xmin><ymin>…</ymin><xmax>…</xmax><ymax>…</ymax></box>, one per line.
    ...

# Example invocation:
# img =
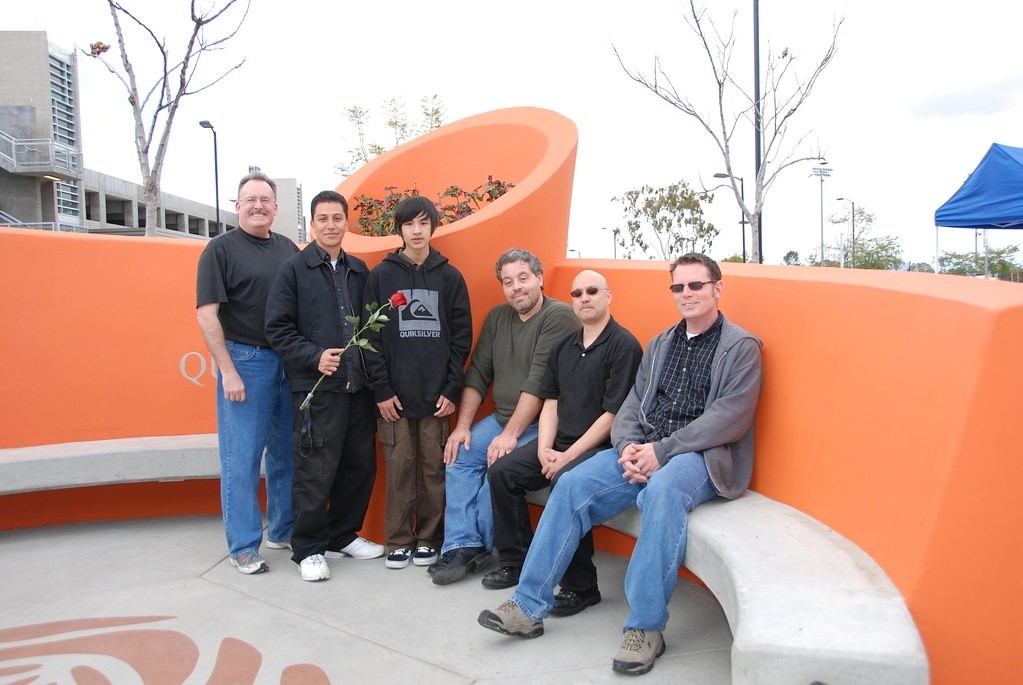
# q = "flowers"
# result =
<box><xmin>301</xmin><ymin>293</ymin><xmax>407</xmax><ymax>410</ymax></box>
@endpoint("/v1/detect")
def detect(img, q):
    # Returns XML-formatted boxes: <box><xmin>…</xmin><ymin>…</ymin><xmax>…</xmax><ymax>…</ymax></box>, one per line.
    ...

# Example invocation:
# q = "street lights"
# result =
<box><xmin>569</xmin><ymin>250</ymin><xmax>580</xmax><ymax>258</ymax></box>
<box><xmin>837</xmin><ymin>198</ymin><xmax>855</xmax><ymax>269</ymax></box>
<box><xmin>713</xmin><ymin>173</ymin><xmax>745</xmax><ymax>264</ymax></box>
<box><xmin>602</xmin><ymin>227</ymin><xmax>616</xmax><ymax>259</ymax></box>
<box><xmin>199</xmin><ymin>120</ymin><xmax>219</xmax><ymax>235</ymax></box>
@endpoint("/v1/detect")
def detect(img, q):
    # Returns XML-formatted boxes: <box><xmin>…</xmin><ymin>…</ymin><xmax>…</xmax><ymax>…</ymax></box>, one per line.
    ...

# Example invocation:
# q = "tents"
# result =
<box><xmin>933</xmin><ymin>142</ymin><xmax>1023</xmax><ymax>283</ymax></box>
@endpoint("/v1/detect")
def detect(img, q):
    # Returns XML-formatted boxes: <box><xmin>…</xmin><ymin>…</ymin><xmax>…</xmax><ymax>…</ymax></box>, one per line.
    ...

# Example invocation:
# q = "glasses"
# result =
<box><xmin>570</xmin><ymin>288</ymin><xmax>609</xmax><ymax>298</ymax></box>
<box><xmin>239</xmin><ymin>198</ymin><xmax>275</xmax><ymax>205</ymax></box>
<box><xmin>670</xmin><ymin>280</ymin><xmax>717</xmax><ymax>293</ymax></box>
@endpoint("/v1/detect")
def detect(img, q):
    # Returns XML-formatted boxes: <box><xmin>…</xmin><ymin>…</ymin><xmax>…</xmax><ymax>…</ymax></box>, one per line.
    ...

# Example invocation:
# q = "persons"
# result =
<box><xmin>478</xmin><ymin>251</ymin><xmax>762</xmax><ymax>676</ymax></box>
<box><xmin>427</xmin><ymin>248</ymin><xmax>580</xmax><ymax>586</ymax></box>
<box><xmin>480</xmin><ymin>270</ymin><xmax>643</xmax><ymax>619</ymax></box>
<box><xmin>197</xmin><ymin>173</ymin><xmax>294</xmax><ymax>573</ymax></box>
<box><xmin>263</xmin><ymin>190</ymin><xmax>384</xmax><ymax>582</ymax></box>
<box><xmin>361</xmin><ymin>196</ymin><xmax>473</xmax><ymax>567</ymax></box>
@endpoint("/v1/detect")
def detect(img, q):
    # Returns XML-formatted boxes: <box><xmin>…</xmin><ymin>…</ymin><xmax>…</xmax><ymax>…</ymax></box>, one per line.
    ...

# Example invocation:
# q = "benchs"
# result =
<box><xmin>0</xmin><ymin>432</ymin><xmax>930</xmax><ymax>685</ymax></box>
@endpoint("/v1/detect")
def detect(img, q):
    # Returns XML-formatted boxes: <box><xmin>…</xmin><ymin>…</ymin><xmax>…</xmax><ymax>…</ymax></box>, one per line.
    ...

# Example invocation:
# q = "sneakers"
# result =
<box><xmin>413</xmin><ymin>547</ymin><xmax>438</xmax><ymax>565</ymax></box>
<box><xmin>385</xmin><ymin>548</ymin><xmax>415</xmax><ymax>568</ymax></box>
<box><xmin>613</xmin><ymin>628</ymin><xmax>665</xmax><ymax>674</ymax></box>
<box><xmin>231</xmin><ymin>552</ymin><xmax>269</xmax><ymax>574</ymax></box>
<box><xmin>478</xmin><ymin>600</ymin><xmax>544</xmax><ymax>638</ymax></box>
<box><xmin>298</xmin><ymin>552</ymin><xmax>330</xmax><ymax>580</ymax></box>
<box><xmin>324</xmin><ymin>536</ymin><xmax>385</xmax><ymax>559</ymax></box>
<box><xmin>266</xmin><ymin>537</ymin><xmax>293</xmax><ymax>552</ymax></box>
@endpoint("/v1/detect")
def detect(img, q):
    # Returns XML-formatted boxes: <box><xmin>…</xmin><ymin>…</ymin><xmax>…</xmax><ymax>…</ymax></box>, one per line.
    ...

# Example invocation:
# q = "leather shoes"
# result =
<box><xmin>550</xmin><ymin>584</ymin><xmax>601</xmax><ymax>616</ymax></box>
<box><xmin>482</xmin><ymin>566</ymin><xmax>523</xmax><ymax>589</ymax></box>
<box><xmin>427</xmin><ymin>546</ymin><xmax>493</xmax><ymax>585</ymax></box>
